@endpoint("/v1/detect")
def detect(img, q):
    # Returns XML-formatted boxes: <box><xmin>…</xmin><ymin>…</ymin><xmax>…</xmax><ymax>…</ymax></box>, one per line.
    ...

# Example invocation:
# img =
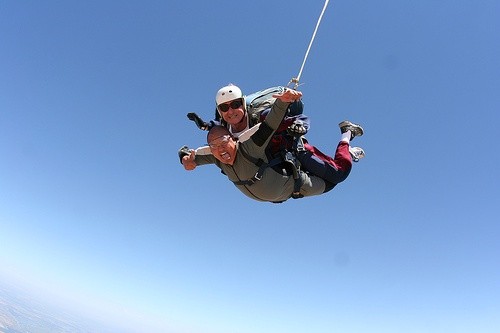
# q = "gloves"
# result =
<box><xmin>187</xmin><ymin>113</ymin><xmax>207</xmax><ymax>130</ymax></box>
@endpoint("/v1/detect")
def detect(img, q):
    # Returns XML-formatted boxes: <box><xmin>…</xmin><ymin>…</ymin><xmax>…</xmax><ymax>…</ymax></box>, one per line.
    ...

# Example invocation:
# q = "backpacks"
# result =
<box><xmin>244</xmin><ymin>86</ymin><xmax>304</xmax><ymax>119</ymax></box>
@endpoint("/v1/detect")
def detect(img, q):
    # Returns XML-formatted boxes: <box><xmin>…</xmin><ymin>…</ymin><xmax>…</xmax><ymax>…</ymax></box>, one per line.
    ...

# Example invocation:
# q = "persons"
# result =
<box><xmin>178</xmin><ymin>89</ymin><xmax>365</xmax><ymax>204</ymax></box>
<box><xmin>187</xmin><ymin>83</ymin><xmax>364</xmax><ymax>184</ymax></box>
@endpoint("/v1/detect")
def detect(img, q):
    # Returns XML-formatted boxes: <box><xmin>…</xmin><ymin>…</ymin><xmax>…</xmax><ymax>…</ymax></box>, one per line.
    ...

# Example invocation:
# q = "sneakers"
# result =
<box><xmin>338</xmin><ymin>121</ymin><xmax>364</xmax><ymax>141</ymax></box>
<box><xmin>349</xmin><ymin>146</ymin><xmax>365</xmax><ymax>162</ymax></box>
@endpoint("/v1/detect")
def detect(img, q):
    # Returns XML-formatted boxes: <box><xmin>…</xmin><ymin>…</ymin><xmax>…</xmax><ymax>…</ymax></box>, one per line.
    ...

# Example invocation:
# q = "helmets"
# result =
<box><xmin>206</xmin><ymin>126</ymin><xmax>229</xmax><ymax>144</ymax></box>
<box><xmin>215</xmin><ymin>85</ymin><xmax>242</xmax><ymax>105</ymax></box>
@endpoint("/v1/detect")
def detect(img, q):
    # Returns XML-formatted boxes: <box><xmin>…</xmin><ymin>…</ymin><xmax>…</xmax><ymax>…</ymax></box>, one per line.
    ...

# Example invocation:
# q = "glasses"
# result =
<box><xmin>218</xmin><ymin>99</ymin><xmax>242</xmax><ymax>112</ymax></box>
<box><xmin>209</xmin><ymin>137</ymin><xmax>231</xmax><ymax>150</ymax></box>
